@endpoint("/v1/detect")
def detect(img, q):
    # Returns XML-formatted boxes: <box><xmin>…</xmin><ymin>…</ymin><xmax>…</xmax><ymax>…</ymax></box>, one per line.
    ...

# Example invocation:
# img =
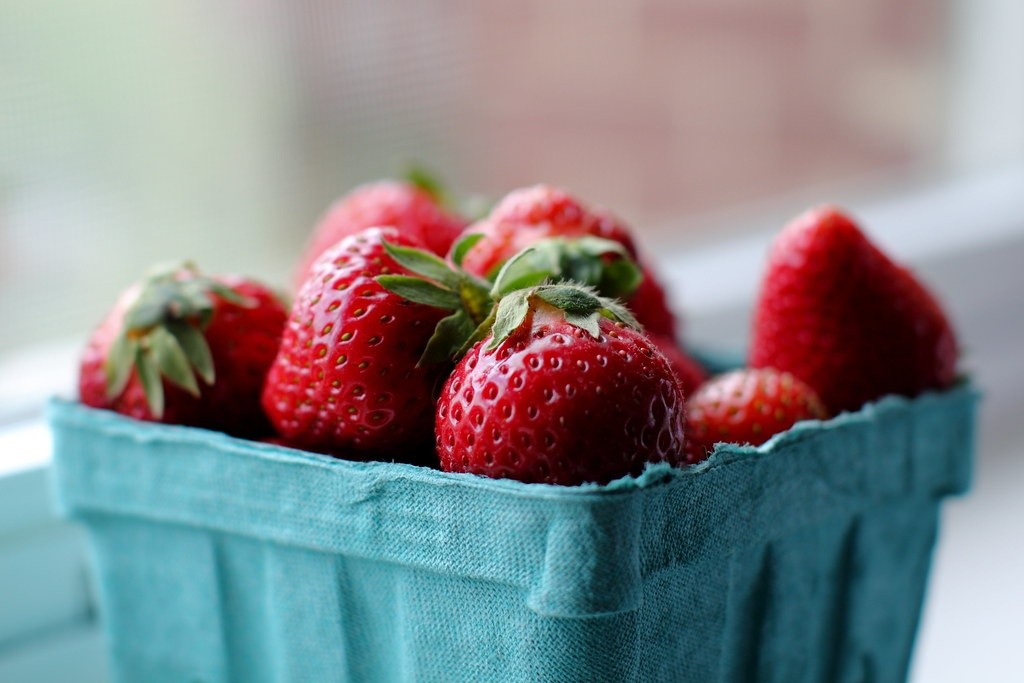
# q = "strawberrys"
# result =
<box><xmin>73</xmin><ymin>167</ymin><xmax>959</xmax><ymax>488</ymax></box>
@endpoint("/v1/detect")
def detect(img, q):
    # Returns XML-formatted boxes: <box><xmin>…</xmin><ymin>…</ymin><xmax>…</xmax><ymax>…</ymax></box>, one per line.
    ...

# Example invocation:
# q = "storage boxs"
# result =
<box><xmin>45</xmin><ymin>345</ymin><xmax>979</xmax><ymax>683</ymax></box>
